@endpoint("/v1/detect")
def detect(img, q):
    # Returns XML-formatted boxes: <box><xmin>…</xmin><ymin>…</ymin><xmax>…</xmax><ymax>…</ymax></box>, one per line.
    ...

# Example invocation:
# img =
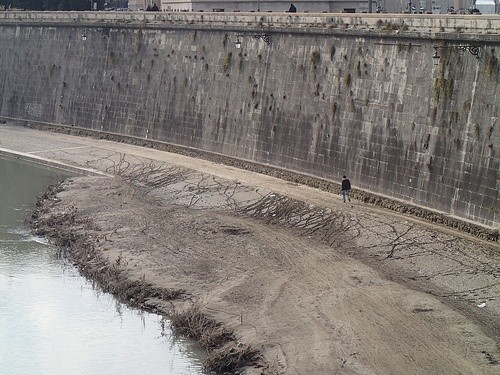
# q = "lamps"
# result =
<box><xmin>234</xmin><ymin>34</ymin><xmax>272</xmax><ymax>50</ymax></box>
<box><xmin>431</xmin><ymin>44</ymin><xmax>480</xmax><ymax>66</ymax></box>
<box><xmin>81</xmin><ymin>27</ymin><xmax>111</xmax><ymax>42</ymax></box>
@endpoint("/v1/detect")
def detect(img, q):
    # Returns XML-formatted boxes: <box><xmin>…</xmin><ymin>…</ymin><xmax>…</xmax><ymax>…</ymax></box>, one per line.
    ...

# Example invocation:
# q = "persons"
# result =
<box><xmin>146</xmin><ymin>5</ymin><xmax>152</xmax><ymax>11</ymax></box>
<box><xmin>151</xmin><ymin>3</ymin><xmax>159</xmax><ymax>11</ymax></box>
<box><xmin>288</xmin><ymin>3</ymin><xmax>296</xmax><ymax>13</ymax></box>
<box><xmin>341</xmin><ymin>175</ymin><xmax>351</xmax><ymax>203</ymax></box>
